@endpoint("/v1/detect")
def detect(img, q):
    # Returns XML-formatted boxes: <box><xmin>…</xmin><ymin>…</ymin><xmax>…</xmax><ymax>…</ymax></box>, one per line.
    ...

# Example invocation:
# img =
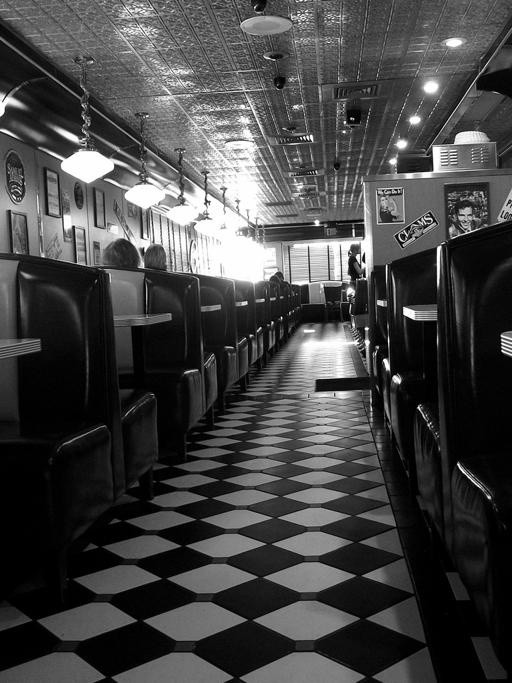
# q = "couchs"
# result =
<box><xmin>300</xmin><ymin>278</ymin><xmax>348</xmax><ymax>322</ymax></box>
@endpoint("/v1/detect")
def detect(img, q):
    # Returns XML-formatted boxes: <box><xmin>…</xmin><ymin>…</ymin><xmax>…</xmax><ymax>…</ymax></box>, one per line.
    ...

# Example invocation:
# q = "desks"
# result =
<box><xmin>349</xmin><ymin>219</ymin><xmax>510</xmax><ymax>670</ymax></box>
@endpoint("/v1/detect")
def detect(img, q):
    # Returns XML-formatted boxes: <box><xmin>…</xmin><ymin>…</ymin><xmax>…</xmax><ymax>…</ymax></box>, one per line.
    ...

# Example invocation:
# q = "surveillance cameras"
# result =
<box><xmin>273</xmin><ymin>77</ymin><xmax>286</xmax><ymax>89</ymax></box>
<box><xmin>333</xmin><ymin>162</ymin><xmax>340</xmax><ymax>171</ymax></box>
<box><xmin>251</xmin><ymin>0</ymin><xmax>268</xmax><ymax>14</ymax></box>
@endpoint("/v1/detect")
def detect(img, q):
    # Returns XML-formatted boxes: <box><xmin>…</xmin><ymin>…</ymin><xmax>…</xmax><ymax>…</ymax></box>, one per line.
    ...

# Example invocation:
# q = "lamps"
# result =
<box><xmin>193</xmin><ymin>171</ymin><xmax>220</xmax><ymax>237</ymax></box>
<box><xmin>216</xmin><ymin>187</ymin><xmax>236</xmax><ymax>243</ymax></box>
<box><xmin>59</xmin><ymin>56</ymin><xmax>114</xmax><ymax>184</ymax></box>
<box><xmin>166</xmin><ymin>148</ymin><xmax>199</xmax><ymax>227</ymax></box>
<box><xmin>124</xmin><ymin>111</ymin><xmax>166</xmax><ymax>210</ymax></box>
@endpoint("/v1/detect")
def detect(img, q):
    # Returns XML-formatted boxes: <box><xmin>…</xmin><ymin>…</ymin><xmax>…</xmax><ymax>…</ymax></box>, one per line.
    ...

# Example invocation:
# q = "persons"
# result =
<box><xmin>348</xmin><ymin>243</ymin><xmax>366</xmax><ymax>283</ymax></box>
<box><xmin>379</xmin><ymin>195</ymin><xmax>399</xmax><ymax>222</ymax></box>
<box><xmin>274</xmin><ymin>271</ymin><xmax>288</xmax><ymax>284</ymax></box>
<box><xmin>144</xmin><ymin>244</ymin><xmax>167</xmax><ymax>271</ymax></box>
<box><xmin>448</xmin><ymin>200</ymin><xmax>482</xmax><ymax>239</ymax></box>
<box><xmin>270</xmin><ymin>275</ymin><xmax>281</xmax><ymax>283</ymax></box>
<box><xmin>102</xmin><ymin>238</ymin><xmax>140</xmax><ymax>268</ymax></box>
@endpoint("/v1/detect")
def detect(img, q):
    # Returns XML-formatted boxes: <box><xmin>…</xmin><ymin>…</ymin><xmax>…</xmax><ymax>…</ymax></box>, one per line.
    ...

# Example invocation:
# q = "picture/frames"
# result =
<box><xmin>44</xmin><ymin>166</ymin><xmax>62</xmax><ymax>218</ymax></box>
<box><xmin>140</xmin><ymin>207</ymin><xmax>149</xmax><ymax>240</ymax></box>
<box><xmin>92</xmin><ymin>187</ymin><xmax>106</xmax><ymax>229</ymax></box>
<box><xmin>8</xmin><ymin>210</ymin><xmax>29</xmax><ymax>255</ymax></box>
<box><xmin>72</xmin><ymin>225</ymin><xmax>90</xmax><ymax>265</ymax></box>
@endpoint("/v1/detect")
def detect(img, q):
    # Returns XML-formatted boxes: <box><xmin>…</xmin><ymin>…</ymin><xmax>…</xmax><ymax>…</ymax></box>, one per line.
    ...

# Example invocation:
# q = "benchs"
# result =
<box><xmin>0</xmin><ymin>257</ymin><xmax>300</xmax><ymax>608</ymax></box>
